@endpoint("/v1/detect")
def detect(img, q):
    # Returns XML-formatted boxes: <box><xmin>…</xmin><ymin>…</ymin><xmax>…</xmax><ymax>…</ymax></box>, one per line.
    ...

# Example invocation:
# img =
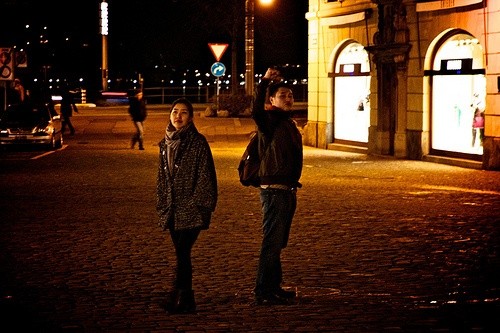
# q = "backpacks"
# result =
<box><xmin>238</xmin><ymin>109</ymin><xmax>274</xmax><ymax>185</ymax></box>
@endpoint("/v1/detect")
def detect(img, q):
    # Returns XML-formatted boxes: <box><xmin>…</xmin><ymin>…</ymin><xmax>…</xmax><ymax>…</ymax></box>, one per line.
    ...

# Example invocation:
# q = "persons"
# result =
<box><xmin>60</xmin><ymin>81</ymin><xmax>78</xmax><ymax>137</ymax></box>
<box><xmin>156</xmin><ymin>98</ymin><xmax>218</xmax><ymax>313</ymax></box>
<box><xmin>128</xmin><ymin>90</ymin><xmax>145</xmax><ymax>150</ymax></box>
<box><xmin>470</xmin><ymin>93</ymin><xmax>485</xmax><ymax>147</ymax></box>
<box><xmin>250</xmin><ymin>67</ymin><xmax>303</xmax><ymax>306</ymax></box>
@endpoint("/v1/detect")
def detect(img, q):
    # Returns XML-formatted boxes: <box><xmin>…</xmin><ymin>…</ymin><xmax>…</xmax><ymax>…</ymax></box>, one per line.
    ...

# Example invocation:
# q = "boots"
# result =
<box><xmin>154</xmin><ymin>289</ymin><xmax>181</xmax><ymax>312</ymax></box>
<box><xmin>167</xmin><ymin>289</ymin><xmax>196</xmax><ymax>314</ymax></box>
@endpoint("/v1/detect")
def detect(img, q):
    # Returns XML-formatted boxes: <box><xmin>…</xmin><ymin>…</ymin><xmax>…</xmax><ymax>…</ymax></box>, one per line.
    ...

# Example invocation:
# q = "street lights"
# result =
<box><xmin>244</xmin><ymin>0</ymin><xmax>272</xmax><ymax>95</ymax></box>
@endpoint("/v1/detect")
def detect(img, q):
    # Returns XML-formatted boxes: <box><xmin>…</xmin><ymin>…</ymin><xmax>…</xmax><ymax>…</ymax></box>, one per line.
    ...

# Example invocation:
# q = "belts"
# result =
<box><xmin>260</xmin><ymin>184</ymin><xmax>295</xmax><ymax>194</ymax></box>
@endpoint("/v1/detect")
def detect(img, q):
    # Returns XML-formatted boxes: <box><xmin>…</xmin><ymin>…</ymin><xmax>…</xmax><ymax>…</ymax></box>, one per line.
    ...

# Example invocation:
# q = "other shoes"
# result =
<box><xmin>139</xmin><ymin>147</ymin><xmax>144</xmax><ymax>150</ymax></box>
<box><xmin>131</xmin><ymin>137</ymin><xmax>134</xmax><ymax>149</ymax></box>
<box><xmin>276</xmin><ymin>286</ymin><xmax>296</xmax><ymax>297</ymax></box>
<box><xmin>255</xmin><ymin>292</ymin><xmax>292</xmax><ymax>306</ymax></box>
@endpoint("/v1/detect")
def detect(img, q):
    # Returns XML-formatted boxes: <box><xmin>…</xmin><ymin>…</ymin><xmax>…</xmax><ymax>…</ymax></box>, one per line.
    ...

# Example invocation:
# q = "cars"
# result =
<box><xmin>0</xmin><ymin>99</ymin><xmax>64</xmax><ymax>151</ymax></box>
